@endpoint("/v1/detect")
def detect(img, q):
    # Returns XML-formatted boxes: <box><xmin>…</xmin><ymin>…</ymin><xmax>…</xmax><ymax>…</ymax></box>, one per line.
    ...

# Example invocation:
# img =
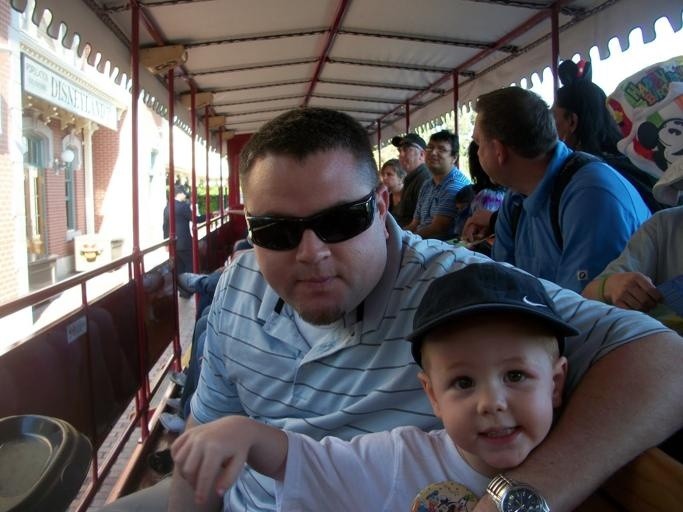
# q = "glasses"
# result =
<box><xmin>425</xmin><ymin>147</ymin><xmax>454</xmax><ymax>153</ymax></box>
<box><xmin>247</xmin><ymin>190</ymin><xmax>379</xmax><ymax>252</ymax></box>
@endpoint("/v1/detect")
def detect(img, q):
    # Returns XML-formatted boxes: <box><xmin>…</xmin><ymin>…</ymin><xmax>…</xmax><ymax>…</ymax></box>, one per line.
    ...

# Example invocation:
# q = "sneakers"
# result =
<box><xmin>170</xmin><ymin>372</ymin><xmax>188</xmax><ymax>387</ymax></box>
<box><xmin>159</xmin><ymin>413</ymin><xmax>185</xmax><ymax>433</ymax></box>
<box><xmin>176</xmin><ymin>272</ymin><xmax>199</xmax><ymax>294</ymax></box>
<box><xmin>166</xmin><ymin>398</ymin><xmax>182</xmax><ymax>409</ymax></box>
<box><xmin>148</xmin><ymin>449</ymin><xmax>173</xmax><ymax>475</ymax></box>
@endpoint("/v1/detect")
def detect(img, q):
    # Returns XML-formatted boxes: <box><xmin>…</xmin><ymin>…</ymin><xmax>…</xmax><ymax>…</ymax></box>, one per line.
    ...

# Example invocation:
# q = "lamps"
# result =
<box><xmin>54</xmin><ymin>150</ymin><xmax>74</xmax><ymax>175</ymax></box>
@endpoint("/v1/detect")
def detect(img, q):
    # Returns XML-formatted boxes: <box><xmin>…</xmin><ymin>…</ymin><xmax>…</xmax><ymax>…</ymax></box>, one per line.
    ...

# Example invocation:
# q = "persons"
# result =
<box><xmin>93</xmin><ymin>58</ymin><xmax>682</xmax><ymax>511</ymax></box>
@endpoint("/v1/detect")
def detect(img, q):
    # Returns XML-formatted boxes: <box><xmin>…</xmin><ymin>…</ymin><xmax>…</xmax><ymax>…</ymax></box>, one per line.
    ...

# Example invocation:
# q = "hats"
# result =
<box><xmin>392</xmin><ymin>133</ymin><xmax>425</xmax><ymax>152</ymax></box>
<box><xmin>404</xmin><ymin>263</ymin><xmax>580</xmax><ymax>368</ymax></box>
<box><xmin>174</xmin><ymin>185</ymin><xmax>186</xmax><ymax>192</ymax></box>
